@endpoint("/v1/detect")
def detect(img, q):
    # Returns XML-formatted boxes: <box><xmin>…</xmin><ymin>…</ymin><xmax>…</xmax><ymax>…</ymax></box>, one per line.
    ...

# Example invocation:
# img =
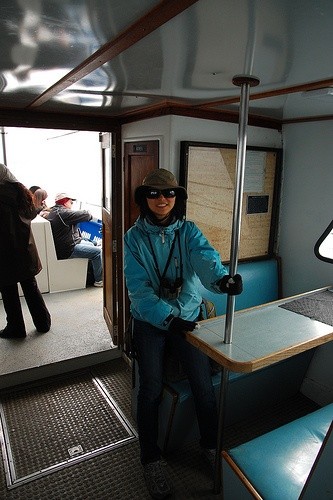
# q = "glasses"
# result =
<box><xmin>146</xmin><ymin>189</ymin><xmax>176</xmax><ymax>199</ymax></box>
<box><xmin>70</xmin><ymin>202</ymin><xmax>73</xmax><ymax>204</ymax></box>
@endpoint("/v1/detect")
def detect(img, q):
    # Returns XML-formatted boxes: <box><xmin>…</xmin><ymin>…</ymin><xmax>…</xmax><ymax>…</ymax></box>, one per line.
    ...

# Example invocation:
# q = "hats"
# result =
<box><xmin>134</xmin><ymin>168</ymin><xmax>189</xmax><ymax>212</ymax></box>
<box><xmin>55</xmin><ymin>193</ymin><xmax>77</xmax><ymax>201</ymax></box>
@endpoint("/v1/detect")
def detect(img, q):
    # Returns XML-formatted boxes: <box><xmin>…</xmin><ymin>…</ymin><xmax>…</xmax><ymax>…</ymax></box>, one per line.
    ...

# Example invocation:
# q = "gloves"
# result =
<box><xmin>170</xmin><ymin>317</ymin><xmax>200</xmax><ymax>334</ymax></box>
<box><xmin>219</xmin><ymin>274</ymin><xmax>243</xmax><ymax>295</ymax></box>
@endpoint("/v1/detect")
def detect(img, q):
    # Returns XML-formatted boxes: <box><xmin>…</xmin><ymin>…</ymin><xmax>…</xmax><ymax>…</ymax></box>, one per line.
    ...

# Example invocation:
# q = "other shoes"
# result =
<box><xmin>0</xmin><ymin>326</ymin><xmax>26</xmax><ymax>339</ymax></box>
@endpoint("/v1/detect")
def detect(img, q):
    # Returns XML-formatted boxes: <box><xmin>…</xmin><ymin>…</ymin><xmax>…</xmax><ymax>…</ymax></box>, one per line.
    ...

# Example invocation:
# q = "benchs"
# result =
<box><xmin>132</xmin><ymin>253</ymin><xmax>317</xmax><ymax>441</ymax></box>
<box><xmin>221</xmin><ymin>403</ymin><xmax>333</xmax><ymax>500</ymax></box>
<box><xmin>0</xmin><ymin>214</ymin><xmax>89</xmax><ymax>300</ymax></box>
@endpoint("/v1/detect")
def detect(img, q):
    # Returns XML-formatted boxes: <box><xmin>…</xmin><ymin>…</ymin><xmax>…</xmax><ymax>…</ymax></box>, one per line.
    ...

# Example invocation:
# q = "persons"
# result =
<box><xmin>122</xmin><ymin>168</ymin><xmax>243</xmax><ymax>500</ymax></box>
<box><xmin>46</xmin><ymin>193</ymin><xmax>103</xmax><ymax>286</ymax></box>
<box><xmin>0</xmin><ymin>165</ymin><xmax>52</xmax><ymax>338</ymax></box>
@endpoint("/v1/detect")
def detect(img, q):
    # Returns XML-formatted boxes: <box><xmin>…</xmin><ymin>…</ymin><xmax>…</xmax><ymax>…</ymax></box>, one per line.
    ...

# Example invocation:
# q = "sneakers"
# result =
<box><xmin>94</xmin><ymin>280</ymin><xmax>104</xmax><ymax>287</ymax></box>
<box><xmin>206</xmin><ymin>447</ymin><xmax>217</xmax><ymax>470</ymax></box>
<box><xmin>141</xmin><ymin>460</ymin><xmax>169</xmax><ymax>496</ymax></box>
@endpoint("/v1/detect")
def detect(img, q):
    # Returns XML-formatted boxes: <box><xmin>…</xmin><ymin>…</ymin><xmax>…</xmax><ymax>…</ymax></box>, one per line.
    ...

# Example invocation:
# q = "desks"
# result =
<box><xmin>183</xmin><ymin>284</ymin><xmax>333</xmax><ymax>494</ymax></box>
